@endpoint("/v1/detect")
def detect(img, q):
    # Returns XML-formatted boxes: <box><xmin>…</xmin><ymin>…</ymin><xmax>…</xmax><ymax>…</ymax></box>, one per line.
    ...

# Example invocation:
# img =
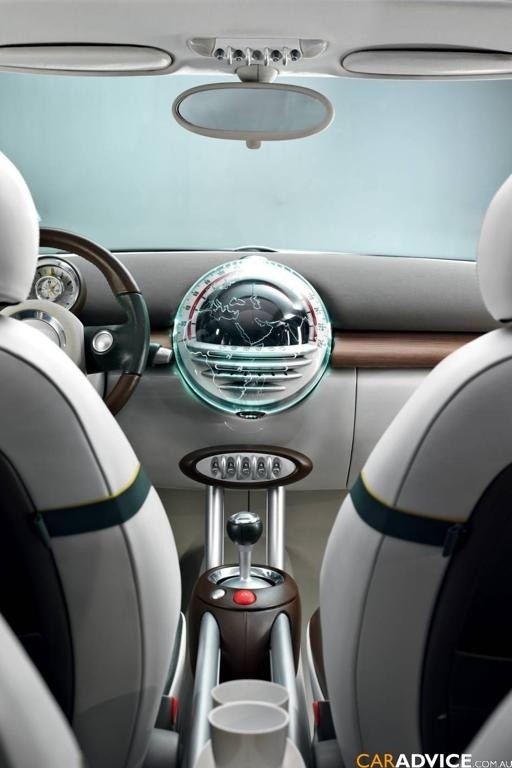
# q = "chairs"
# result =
<box><xmin>302</xmin><ymin>175</ymin><xmax>510</xmax><ymax>767</ymax></box>
<box><xmin>0</xmin><ymin>147</ymin><xmax>189</xmax><ymax>766</ymax></box>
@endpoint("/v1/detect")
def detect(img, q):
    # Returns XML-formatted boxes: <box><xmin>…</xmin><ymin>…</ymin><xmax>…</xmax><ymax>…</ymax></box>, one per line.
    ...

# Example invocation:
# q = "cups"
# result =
<box><xmin>210</xmin><ymin>674</ymin><xmax>289</xmax><ymax>718</ymax></box>
<box><xmin>208</xmin><ymin>704</ymin><xmax>288</xmax><ymax>767</ymax></box>
